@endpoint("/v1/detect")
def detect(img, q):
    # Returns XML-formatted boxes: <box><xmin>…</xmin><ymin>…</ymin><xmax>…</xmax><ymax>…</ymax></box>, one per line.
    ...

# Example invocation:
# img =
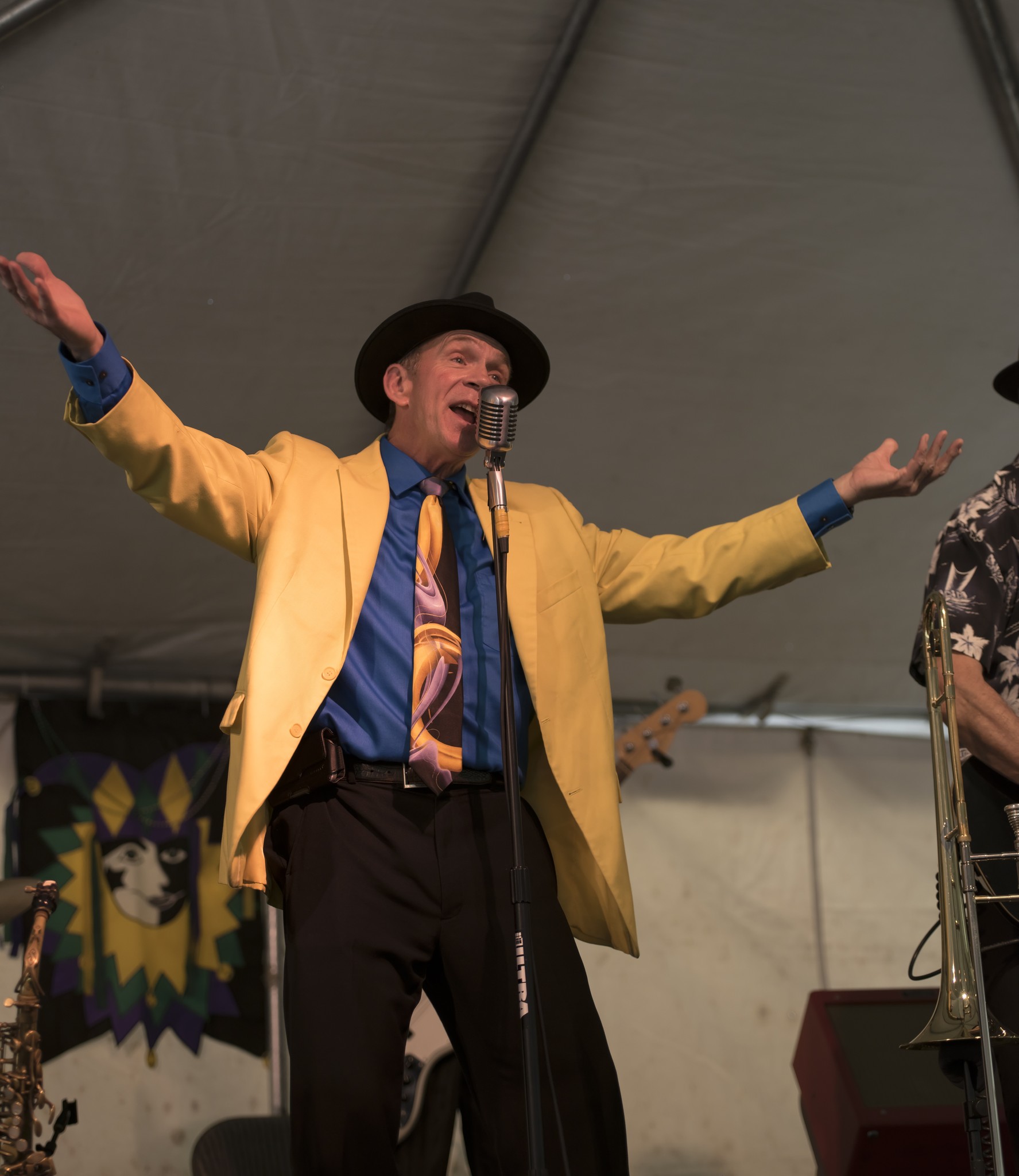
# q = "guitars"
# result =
<box><xmin>614</xmin><ymin>686</ymin><xmax>709</xmax><ymax>790</ymax></box>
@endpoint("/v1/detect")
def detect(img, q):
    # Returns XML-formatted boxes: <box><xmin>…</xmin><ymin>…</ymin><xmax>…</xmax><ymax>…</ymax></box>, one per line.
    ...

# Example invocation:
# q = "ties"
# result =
<box><xmin>408</xmin><ymin>474</ymin><xmax>464</xmax><ymax>796</ymax></box>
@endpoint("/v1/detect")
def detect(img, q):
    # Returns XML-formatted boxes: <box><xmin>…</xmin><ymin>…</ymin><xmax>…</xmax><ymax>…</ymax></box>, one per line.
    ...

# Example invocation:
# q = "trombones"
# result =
<box><xmin>896</xmin><ymin>589</ymin><xmax>1019</xmax><ymax>1176</ymax></box>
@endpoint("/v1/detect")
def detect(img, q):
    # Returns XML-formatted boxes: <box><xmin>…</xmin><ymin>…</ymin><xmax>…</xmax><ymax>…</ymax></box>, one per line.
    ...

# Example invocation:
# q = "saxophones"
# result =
<box><xmin>0</xmin><ymin>878</ymin><xmax>61</xmax><ymax>1176</ymax></box>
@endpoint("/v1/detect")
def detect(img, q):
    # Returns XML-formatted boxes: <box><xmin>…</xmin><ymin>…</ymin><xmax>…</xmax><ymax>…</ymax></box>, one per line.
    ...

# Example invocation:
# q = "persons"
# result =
<box><xmin>0</xmin><ymin>251</ymin><xmax>963</xmax><ymax>1176</ymax></box>
<box><xmin>908</xmin><ymin>361</ymin><xmax>1019</xmax><ymax>1176</ymax></box>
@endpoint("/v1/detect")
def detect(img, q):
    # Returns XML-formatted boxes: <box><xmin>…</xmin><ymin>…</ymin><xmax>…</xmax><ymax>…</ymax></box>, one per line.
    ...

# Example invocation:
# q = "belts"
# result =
<box><xmin>349</xmin><ymin>759</ymin><xmax>505</xmax><ymax>787</ymax></box>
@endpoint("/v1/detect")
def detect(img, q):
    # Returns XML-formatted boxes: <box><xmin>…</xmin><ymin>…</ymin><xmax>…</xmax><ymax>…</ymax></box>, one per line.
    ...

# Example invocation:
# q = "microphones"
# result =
<box><xmin>474</xmin><ymin>384</ymin><xmax>518</xmax><ymax>454</ymax></box>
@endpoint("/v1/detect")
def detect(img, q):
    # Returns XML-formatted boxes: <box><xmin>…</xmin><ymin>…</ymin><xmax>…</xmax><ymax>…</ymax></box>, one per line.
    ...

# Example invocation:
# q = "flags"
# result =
<box><xmin>6</xmin><ymin>682</ymin><xmax>268</xmax><ymax>1063</ymax></box>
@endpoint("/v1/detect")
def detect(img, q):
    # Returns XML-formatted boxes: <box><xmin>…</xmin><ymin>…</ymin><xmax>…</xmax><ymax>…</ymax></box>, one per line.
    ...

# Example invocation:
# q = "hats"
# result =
<box><xmin>354</xmin><ymin>292</ymin><xmax>550</xmax><ymax>429</ymax></box>
<box><xmin>992</xmin><ymin>360</ymin><xmax>1019</xmax><ymax>404</ymax></box>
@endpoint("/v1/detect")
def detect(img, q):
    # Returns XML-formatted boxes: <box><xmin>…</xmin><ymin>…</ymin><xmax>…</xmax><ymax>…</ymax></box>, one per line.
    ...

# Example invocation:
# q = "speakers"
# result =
<box><xmin>793</xmin><ymin>987</ymin><xmax>1016</xmax><ymax>1176</ymax></box>
